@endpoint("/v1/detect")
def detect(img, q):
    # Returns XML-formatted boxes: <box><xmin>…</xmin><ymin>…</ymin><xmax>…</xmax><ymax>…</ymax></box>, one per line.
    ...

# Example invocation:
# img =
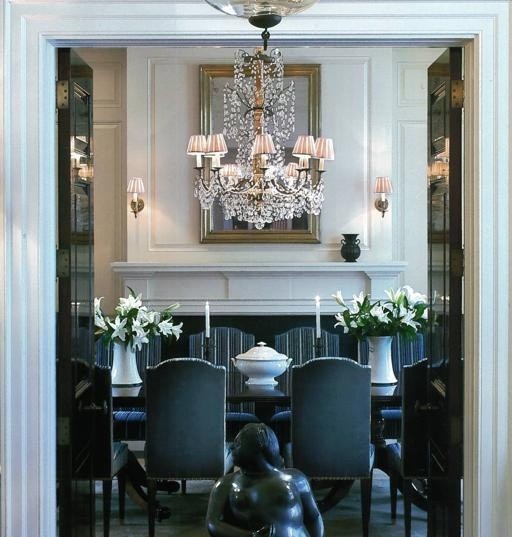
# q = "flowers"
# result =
<box><xmin>87</xmin><ymin>280</ymin><xmax>184</xmax><ymax>350</ymax></box>
<box><xmin>327</xmin><ymin>286</ymin><xmax>431</xmax><ymax>343</ymax></box>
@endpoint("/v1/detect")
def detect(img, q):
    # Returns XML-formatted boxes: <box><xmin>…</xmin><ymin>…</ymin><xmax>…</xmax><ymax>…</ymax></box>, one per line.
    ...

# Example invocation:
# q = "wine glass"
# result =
<box><xmin>231</xmin><ymin>341</ymin><xmax>293</xmax><ymax>386</ymax></box>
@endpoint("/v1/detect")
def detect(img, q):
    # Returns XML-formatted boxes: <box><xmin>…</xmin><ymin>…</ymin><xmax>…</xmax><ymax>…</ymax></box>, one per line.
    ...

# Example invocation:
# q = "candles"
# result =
<box><xmin>204</xmin><ymin>301</ymin><xmax>211</xmax><ymax>337</ymax></box>
<box><xmin>315</xmin><ymin>296</ymin><xmax>321</xmax><ymax>339</ymax></box>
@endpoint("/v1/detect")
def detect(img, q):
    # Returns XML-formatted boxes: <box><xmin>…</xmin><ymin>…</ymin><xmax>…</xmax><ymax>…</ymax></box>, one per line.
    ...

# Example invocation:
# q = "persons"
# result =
<box><xmin>206</xmin><ymin>420</ymin><xmax>324</xmax><ymax>537</ymax></box>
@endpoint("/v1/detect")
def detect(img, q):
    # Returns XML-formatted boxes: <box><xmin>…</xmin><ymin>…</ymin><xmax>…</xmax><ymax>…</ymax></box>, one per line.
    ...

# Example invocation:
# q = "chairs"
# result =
<box><xmin>73</xmin><ymin>327</ymin><xmax>430</xmax><ymax>536</ymax></box>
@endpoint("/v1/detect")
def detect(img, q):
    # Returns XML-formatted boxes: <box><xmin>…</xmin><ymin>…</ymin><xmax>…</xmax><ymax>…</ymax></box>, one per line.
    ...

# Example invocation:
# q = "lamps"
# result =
<box><xmin>373</xmin><ymin>175</ymin><xmax>394</xmax><ymax>218</ymax></box>
<box><xmin>203</xmin><ymin>0</ymin><xmax>320</xmax><ymax>53</ymax></box>
<box><xmin>123</xmin><ymin>176</ymin><xmax>147</xmax><ymax>219</ymax></box>
<box><xmin>189</xmin><ymin>44</ymin><xmax>335</xmax><ymax>233</ymax></box>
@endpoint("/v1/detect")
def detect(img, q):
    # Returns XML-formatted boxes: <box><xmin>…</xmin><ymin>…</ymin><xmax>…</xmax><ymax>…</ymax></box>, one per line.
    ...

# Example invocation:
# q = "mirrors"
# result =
<box><xmin>195</xmin><ymin>60</ymin><xmax>326</xmax><ymax>243</ymax></box>
